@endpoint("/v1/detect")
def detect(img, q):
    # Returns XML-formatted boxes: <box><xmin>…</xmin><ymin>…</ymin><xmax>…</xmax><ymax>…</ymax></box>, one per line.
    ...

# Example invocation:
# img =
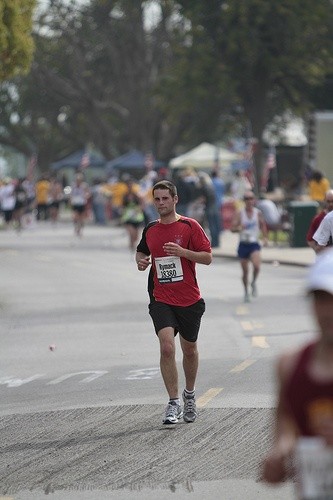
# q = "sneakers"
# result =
<box><xmin>182</xmin><ymin>389</ymin><xmax>197</xmax><ymax>423</ymax></box>
<box><xmin>162</xmin><ymin>400</ymin><xmax>183</xmax><ymax>424</ymax></box>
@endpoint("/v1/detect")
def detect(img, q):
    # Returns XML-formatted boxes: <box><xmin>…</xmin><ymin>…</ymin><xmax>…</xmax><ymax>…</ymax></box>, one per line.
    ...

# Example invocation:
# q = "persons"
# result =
<box><xmin>134</xmin><ymin>181</ymin><xmax>213</xmax><ymax>424</ymax></box>
<box><xmin>1</xmin><ymin>159</ymin><xmax>333</xmax><ymax>305</ymax></box>
<box><xmin>259</xmin><ymin>247</ymin><xmax>333</xmax><ymax>500</ymax></box>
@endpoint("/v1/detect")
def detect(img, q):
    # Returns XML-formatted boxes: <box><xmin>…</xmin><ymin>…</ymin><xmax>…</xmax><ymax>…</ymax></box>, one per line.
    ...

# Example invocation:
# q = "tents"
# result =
<box><xmin>48</xmin><ymin>142</ymin><xmax>245</xmax><ymax>170</ymax></box>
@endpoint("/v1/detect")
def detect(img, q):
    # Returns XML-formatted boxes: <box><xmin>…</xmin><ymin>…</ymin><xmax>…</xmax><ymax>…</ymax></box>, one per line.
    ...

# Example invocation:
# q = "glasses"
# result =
<box><xmin>244</xmin><ymin>196</ymin><xmax>253</xmax><ymax>201</ymax></box>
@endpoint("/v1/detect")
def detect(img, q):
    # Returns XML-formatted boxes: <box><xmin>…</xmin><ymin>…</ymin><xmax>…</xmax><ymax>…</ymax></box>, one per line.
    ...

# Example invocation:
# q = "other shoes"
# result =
<box><xmin>244</xmin><ymin>282</ymin><xmax>258</xmax><ymax>303</ymax></box>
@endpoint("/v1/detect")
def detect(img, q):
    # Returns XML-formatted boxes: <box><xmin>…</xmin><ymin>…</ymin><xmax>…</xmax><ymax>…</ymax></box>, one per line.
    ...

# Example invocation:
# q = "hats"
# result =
<box><xmin>305</xmin><ymin>251</ymin><xmax>333</xmax><ymax>296</ymax></box>
